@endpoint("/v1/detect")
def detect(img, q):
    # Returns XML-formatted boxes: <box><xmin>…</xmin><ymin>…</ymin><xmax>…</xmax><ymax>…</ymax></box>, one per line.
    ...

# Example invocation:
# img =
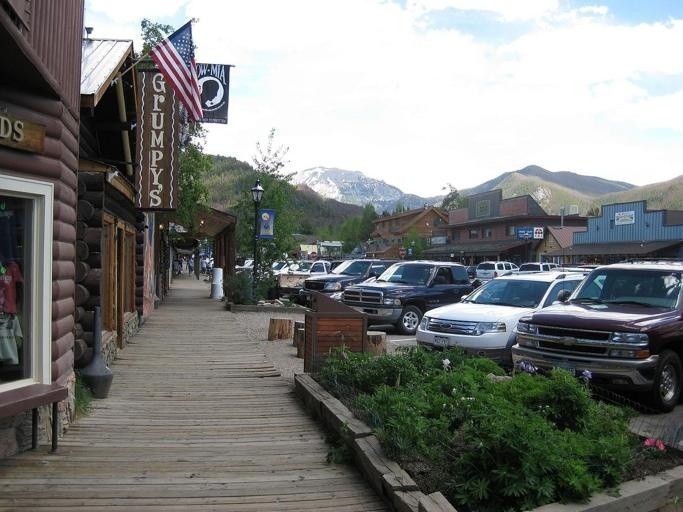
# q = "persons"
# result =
<box><xmin>178</xmin><ymin>254</ymin><xmax>207</xmax><ymax>275</ymax></box>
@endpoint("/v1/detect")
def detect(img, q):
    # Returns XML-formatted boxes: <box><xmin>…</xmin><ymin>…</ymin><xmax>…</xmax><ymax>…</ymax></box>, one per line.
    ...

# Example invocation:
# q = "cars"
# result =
<box><xmin>236</xmin><ymin>257</ymin><xmax>331</xmax><ymax>276</ymax></box>
<box><xmin>416</xmin><ymin>270</ymin><xmax>588</xmax><ymax>365</ymax></box>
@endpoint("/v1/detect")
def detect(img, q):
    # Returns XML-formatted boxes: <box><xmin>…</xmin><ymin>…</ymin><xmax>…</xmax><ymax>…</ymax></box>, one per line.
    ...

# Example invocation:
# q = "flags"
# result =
<box><xmin>149</xmin><ymin>22</ymin><xmax>204</xmax><ymax>122</ymax></box>
<box><xmin>190</xmin><ymin>63</ymin><xmax>228</xmax><ymax>125</ymax></box>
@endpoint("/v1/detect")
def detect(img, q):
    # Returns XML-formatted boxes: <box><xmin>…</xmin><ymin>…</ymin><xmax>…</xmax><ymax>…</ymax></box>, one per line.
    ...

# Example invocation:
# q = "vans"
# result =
<box><xmin>476</xmin><ymin>261</ymin><xmax>559</xmax><ymax>285</ymax></box>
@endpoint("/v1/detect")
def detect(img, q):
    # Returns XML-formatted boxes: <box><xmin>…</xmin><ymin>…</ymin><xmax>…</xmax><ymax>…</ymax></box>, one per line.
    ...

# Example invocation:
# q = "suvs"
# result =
<box><xmin>302</xmin><ymin>257</ymin><xmax>474</xmax><ymax>335</ymax></box>
<box><xmin>511</xmin><ymin>258</ymin><xmax>683</xmax><ymax>411</ymax></box>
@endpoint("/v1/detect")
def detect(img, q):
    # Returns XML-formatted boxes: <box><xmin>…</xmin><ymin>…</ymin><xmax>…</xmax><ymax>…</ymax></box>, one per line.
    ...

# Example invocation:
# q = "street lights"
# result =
<box><xmin>250</xmin><ymin>178</ymin><xmax>265</xmax><ymax>304</ymax></box>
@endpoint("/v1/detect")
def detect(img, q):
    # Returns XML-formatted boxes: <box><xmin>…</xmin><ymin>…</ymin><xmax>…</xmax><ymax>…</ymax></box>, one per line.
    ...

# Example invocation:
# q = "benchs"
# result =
<box><xmin>0</xmin><ymin>383</ymin><xmax>68</xmax><ymax>455</ymax></box>
